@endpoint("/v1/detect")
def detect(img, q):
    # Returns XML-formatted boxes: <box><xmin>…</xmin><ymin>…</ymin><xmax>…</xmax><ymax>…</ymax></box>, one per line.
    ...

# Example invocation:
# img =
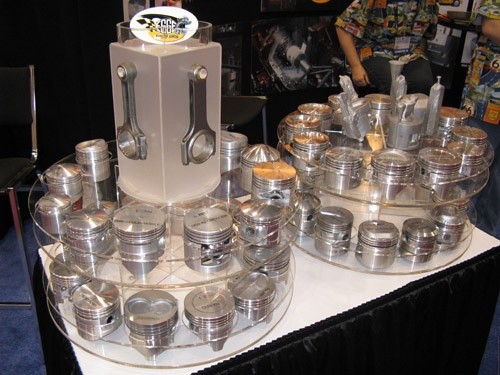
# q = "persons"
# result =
<box><xmin>334</xmin><ymin>0</ymin><xmax>441</xmax><ymax>97</ymax></box>
<box><xmin>460</xmin><ymin>0</ymin><xmax>500</xmax><ymax>239</ymax></box>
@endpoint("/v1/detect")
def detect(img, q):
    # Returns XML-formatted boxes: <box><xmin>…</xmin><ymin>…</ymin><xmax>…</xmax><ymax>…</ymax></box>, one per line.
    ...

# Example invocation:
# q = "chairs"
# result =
<box><xmin>0</xmin><ymin>65</ymin><xmax>48</xmax><ymax>286</ymax></box>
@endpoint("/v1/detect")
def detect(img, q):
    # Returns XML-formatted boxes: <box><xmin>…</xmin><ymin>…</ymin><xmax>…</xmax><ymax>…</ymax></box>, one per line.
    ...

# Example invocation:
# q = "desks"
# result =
<box><xmin>33</xmin><ymin>178</ymin><xmax>500</xmax><ymax>375</ymax></box>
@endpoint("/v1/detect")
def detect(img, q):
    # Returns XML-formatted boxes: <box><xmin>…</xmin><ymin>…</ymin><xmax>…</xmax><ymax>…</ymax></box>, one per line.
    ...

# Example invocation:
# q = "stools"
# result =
<box><xmin>221</xmin><ymin>95</ymin><xmax>268</xmax><ymax>146</ymax></box>
